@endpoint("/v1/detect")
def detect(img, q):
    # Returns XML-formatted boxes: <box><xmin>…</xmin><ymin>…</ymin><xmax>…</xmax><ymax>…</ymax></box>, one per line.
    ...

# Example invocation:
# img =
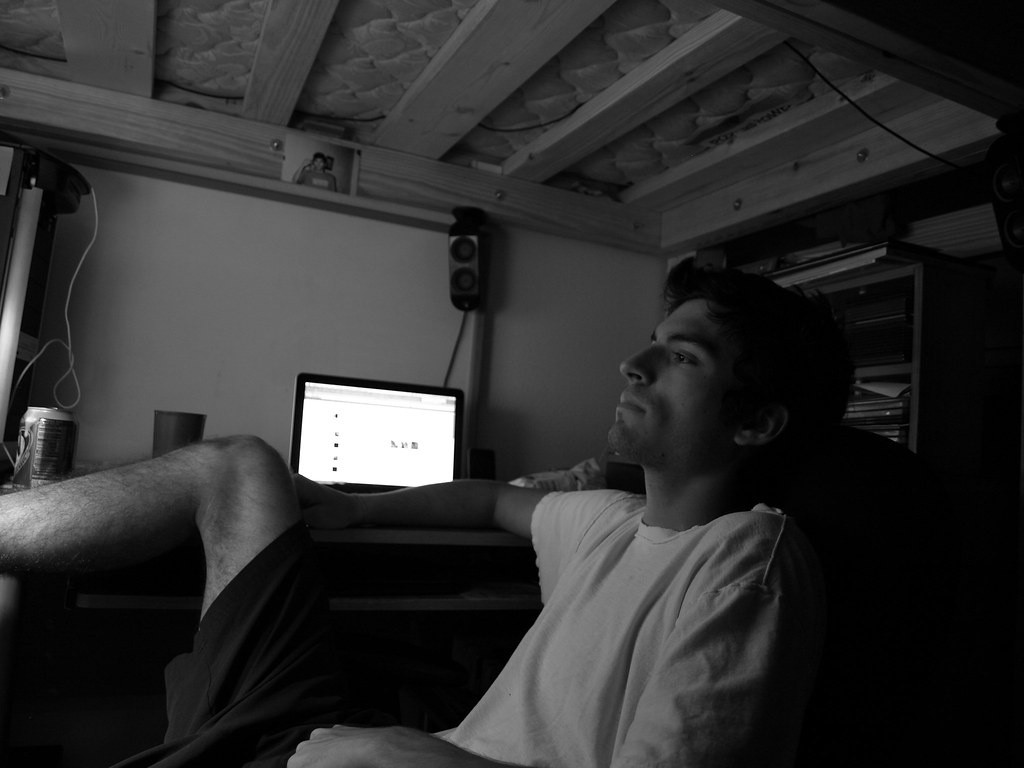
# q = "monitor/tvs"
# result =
<box><xmin>289</xmin><ymin>373</ymin><xmax>465</xmax><ymax>494</ymax></box>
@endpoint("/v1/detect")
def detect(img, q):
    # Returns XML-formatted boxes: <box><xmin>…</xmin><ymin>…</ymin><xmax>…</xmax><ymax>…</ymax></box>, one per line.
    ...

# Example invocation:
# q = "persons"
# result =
<box><xmin>296</xmin><ymin>153</ymin><xmax>337</xmax><ymax>193</ymax></box>
<box><xmin>0</xmin><ymin>260</ymin><xmax>861</xmax><ymax>768</ymax></box>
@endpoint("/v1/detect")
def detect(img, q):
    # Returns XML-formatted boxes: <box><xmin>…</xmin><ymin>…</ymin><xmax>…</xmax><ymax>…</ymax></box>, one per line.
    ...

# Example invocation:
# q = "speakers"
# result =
<box><xmin>448</xmin><ymin>222</ymin><xmax>486</xmax><ymax>310</ymax></box>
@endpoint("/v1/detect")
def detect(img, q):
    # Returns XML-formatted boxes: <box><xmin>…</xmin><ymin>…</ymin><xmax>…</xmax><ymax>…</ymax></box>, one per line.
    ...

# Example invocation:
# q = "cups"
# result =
<box><xmin>152</xmin><ymin>410</ymin><xmax>207</xmax><ymax>459</ymax></box>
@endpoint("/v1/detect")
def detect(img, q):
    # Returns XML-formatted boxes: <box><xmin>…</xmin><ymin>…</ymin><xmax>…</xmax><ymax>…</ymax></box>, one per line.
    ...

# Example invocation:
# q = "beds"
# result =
<box><xmin>0</xmin><ymin>0</ymin><xmax>1024</xmax><ymax>275</ymax></box>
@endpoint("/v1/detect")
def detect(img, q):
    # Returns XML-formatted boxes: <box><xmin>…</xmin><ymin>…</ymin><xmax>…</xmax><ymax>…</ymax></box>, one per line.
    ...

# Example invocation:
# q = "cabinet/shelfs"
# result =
<box><xmin>796</xmin><ymin>261</ymin><xmax>987</xmax><ymax>460</ymax></box>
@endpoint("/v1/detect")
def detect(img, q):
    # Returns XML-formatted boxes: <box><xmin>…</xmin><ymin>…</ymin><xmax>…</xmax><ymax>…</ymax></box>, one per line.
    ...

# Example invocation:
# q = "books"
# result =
<box><xmin>760</xmin><ymin>241</ymin><xmax>963</xmax><ymax>446</ymax></box>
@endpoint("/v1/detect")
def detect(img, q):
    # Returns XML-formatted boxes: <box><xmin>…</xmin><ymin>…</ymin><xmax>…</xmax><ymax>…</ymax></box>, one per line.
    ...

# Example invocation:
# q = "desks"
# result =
<box><xmin>72</xmin><ymin>525</ymin><xmax>545</xmax><ymax>620</ymax></box>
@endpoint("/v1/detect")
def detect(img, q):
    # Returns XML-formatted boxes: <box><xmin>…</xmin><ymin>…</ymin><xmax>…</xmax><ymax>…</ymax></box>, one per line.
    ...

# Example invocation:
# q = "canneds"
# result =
<box><xmin>12</xmin><ymin>407</ymin><xmax>79</xmax><ymax>492</ymax></box>
